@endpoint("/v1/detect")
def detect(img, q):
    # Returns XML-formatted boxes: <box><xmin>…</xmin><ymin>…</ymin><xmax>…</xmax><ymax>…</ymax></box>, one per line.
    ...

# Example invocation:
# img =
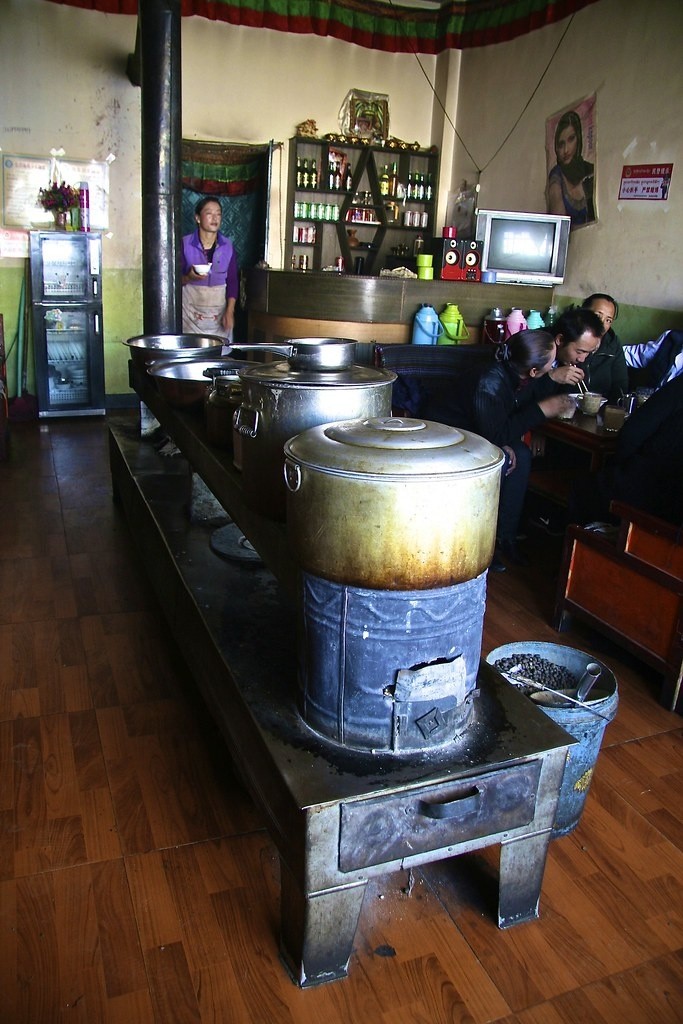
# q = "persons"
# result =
<box><xmin>415</xmin><ymin>293</ymin><xmax>683</xmax><ymax>574</ymax></box>
<box><xmin>179</xmin><ymin>198</ymin><xmax>239</xmax><ymax>356</ymax></box>
<box><xmin>548</xmin><ymin>111</ymin><xmax>595</xmax><ymax>224</ymax></box>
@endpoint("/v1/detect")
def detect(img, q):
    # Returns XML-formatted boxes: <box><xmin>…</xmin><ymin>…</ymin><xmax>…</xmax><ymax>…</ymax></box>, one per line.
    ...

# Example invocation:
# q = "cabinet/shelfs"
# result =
<box><xmin>284</xmin><ymin>135</ymin><xmax>442</xmax><ymax>274</ymax></box>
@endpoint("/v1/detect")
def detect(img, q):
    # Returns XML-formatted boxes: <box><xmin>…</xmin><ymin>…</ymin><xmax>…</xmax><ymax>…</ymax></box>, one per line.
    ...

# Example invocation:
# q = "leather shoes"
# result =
<box><xmin>491</xmin><ymin>559</ymin><xmax>508</xmax><ymax>573</ymax></box>
<box><xmin>504</xmin><ymin>552</ymin><xmax>530</xmax><ymax>567</ymax></box>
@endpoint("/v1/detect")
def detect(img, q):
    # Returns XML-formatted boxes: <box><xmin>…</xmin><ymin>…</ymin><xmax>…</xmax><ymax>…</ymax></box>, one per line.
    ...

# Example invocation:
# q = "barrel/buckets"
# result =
<box><xmin>486</xmin><ymin>641</ymin><xmax>620</xmax><ymax>838</ymax></box>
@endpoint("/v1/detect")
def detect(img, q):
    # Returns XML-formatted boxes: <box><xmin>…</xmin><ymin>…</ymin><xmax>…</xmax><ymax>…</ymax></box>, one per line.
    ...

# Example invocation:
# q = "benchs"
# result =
<box><xmin>555</xmin><ymin>499</ymin><xmax>683</xmax><ymax>713</ymax></box>
<box><xmin>375</xmin><ymin>340</ymin><xmax>498</xmax><ymax>419</ymax></box>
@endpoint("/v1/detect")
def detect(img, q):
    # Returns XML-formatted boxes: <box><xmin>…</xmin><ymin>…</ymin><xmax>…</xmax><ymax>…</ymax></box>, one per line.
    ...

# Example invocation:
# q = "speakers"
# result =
<box><xmin>430</xmin><ymin>237</ymin><xmax>462</xmax><ymax>280</ymax></box>
<box><xmin>461</xmin><ymin>240</ymin><xmax>484</xmax><ymax>282</ymax></box>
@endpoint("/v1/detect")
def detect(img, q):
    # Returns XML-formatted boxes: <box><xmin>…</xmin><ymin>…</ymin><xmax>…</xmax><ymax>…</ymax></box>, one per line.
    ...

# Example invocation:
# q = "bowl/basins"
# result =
<box><xmin>56</xmin><ymin>365</ymin><xmax>88</xmax><ymax>389</ymax></box>
<box><xmin>568</xmin><ymin>393</ymin><xmax>607</xmax><ymax>410</ymax></box>
<box><xmin>193</xmin><ymin>265</ymin><xmax>211</xmax><ymax>276</ymax></box>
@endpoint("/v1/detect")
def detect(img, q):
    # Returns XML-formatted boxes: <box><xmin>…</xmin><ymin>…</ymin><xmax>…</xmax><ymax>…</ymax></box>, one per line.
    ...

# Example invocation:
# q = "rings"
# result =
<box><xmin>537</xmin><ymin>448</ymin><xmax>541</xmax><ymax>452</ymax></box>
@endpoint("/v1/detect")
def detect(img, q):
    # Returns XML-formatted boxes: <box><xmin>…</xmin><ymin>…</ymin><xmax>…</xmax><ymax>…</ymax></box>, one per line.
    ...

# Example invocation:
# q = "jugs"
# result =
<box><xmin>438</xmin><ymin>302</ymin><xmax>470</xmax><ymax>345</ymax></box>
<box><xmin>411</xmin><ymin>303</ymin><xmax>445</xmax><ymax>345</ymax></box>
<box><xmin>543</xmin><ymin>309</ymin><xmax>557</xmax><ymax>328</ymax></box>
<box><xmin>526</xmin><ymin>309</ymin><xmax>546</xmax><ymax>330</ymax></box>
<box><xmin>481</xmin><ymin>307</ymin><xmax>507</xmax><ymax>346</ymax></box>
<box><xmin>506</xmin><ymin>307</ymin><xmax>528</xmax><ymax>335</ymax></box>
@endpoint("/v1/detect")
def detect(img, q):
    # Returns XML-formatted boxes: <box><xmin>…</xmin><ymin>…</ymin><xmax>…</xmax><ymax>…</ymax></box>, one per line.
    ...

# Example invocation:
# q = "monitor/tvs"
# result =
<box><xmin>475</xmin><ymin>209</ymin><xmax>571</xmax><ymax>284</ymax></box>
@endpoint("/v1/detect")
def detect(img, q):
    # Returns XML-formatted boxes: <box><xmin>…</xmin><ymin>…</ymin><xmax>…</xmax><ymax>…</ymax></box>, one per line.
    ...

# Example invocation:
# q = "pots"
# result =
<box><xmin>126</xmin><ymin>333</ymin><xmax>516</xmax><ymax>590</ymax></box>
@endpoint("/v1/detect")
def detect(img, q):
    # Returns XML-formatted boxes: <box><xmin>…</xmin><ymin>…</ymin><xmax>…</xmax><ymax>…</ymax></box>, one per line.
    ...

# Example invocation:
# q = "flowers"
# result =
<box><xmin>38</xmin><ymin>179</ymin><xmax>79</xmax><ymax>213</ymax></box>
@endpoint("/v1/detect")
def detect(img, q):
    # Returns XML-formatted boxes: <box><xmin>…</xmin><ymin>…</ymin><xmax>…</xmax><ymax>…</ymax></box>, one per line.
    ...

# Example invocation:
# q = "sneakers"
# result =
<box><xmin>529</xmin><ymin>510</ymin><xmax>564</xmax><ymax>535</ymax></box>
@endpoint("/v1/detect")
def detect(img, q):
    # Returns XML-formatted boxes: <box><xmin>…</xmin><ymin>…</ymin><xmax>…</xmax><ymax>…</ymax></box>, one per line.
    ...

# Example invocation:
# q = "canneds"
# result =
<box><xmin>343</xmin><ymin>207</ymin><xmax>375</xmax><ymax>221</ymax></box>
<box><xmin>292</xmin><ymin>254</ymin><xmax>308</xmax><ymax>270</ymax></box>
<box><xmin>404</xmin><ymin>210</ymin><xmax>428</xmax><ymax>228</ymax></box>
<box><xmin>335</xmin><ymin>256</ymin><xmax>345</xmax><ymax>272</ymax></box>
<box><xmin>294</xmin><ymin>201</ymin><xmax>339</xmax><ymax>221</ymax></box>
<box><xmin>293</xmin><ymin>225</ymin><xmax>316</xmax><ymax>244</ymax></box>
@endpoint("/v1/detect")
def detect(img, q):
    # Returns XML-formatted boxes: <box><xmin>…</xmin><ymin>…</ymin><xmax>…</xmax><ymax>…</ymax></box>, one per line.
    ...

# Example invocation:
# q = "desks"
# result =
<box><xmin>522</xmin><ymin>415</ymin><xmax>619</xmax><ymax>514</ymax></box>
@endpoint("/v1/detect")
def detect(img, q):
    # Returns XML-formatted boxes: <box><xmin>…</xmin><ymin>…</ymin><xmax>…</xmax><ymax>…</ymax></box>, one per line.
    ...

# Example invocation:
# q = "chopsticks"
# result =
<box><xmin>569</xmin><ymin>363</ymin><xmax>589</xmax><ymax>395</ymax></box>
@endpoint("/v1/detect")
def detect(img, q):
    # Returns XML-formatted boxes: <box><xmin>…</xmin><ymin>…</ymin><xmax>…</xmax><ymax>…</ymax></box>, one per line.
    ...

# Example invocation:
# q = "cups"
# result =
<box><xmin>618</xmin><ymin>393</ymin><xmax>639</xmax><ymax>418</ymax></box>
<box><xmin>604</xmin><ymin>404</ymin><xmax>624</xmax><ymax>432</ymax></box>
<box><xmin>576</xmin><ymin>392</ymin><xmax>602</xmax><ymax>417</ymax></box>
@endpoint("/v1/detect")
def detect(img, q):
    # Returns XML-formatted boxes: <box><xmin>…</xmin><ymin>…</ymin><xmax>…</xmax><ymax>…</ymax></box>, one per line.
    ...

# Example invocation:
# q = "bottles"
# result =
<box><xmin>354</xmin><ymin>257</ymin><xmax>365</xmax><ymax>275</ymax></box>
<box><xmin>379</xmin><ymin>162</ymin><xmax>436</xmax><ymax>201</ymax></box>
<box><xmin>327</xmin><ymin>162</ymin><xmax>353</xmax><ymax>192</ymax></box>
<box><xmin>296</xmin><ymin>157</ymin><xmax>318</xmax><ymax>191</ymax></box>
<box><xmin>80</xmin><ymin>182</ymin><xmax>90</xmax><ymax>232</ymax></box>
<box><xmin>413</xmin><ymin>236</ymin><xmax>425</xmax><ymax>257</ymax></box>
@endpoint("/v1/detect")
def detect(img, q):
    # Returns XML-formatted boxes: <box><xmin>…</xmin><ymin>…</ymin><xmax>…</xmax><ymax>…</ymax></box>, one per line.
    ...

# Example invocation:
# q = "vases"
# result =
<box><xmin>55</xmin><ymin>209</ymin><xmax>66</xmax><ymax>230</ymax></box>
<box><xmin>347</xmin><ymin>228</ymin><xmax>359</xmax><ymax>247</ymax></box>
